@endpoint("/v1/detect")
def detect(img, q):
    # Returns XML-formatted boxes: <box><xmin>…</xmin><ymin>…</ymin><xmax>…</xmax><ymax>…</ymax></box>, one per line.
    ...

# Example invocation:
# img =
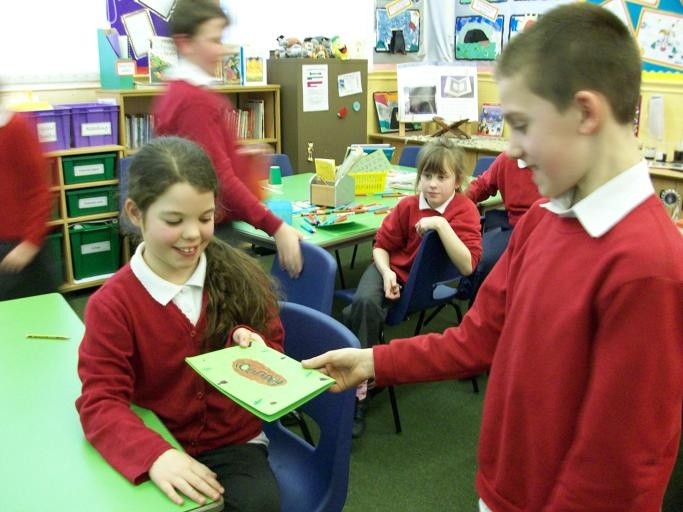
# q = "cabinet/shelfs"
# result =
<box><xmin>38</xmin><ymin>146</ymin><xmax>127</xmax><ymax>293</ymax></box>
<box><xmin>96</xmin><ymin>83</ymin><xmax>285</xmax><ymax>175</ymax></box>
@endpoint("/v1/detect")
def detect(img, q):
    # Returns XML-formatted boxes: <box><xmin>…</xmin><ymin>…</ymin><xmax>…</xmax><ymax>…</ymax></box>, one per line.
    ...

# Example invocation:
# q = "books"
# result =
<box><xmin>125</xmin><ymin>98</ymin><xmax>267</xmax><ymax>152</ymax></box>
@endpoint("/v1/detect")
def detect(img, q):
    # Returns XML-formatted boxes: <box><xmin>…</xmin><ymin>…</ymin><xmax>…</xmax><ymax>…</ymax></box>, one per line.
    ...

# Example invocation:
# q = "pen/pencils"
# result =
<box><xmin>27</xmin><ymin>336</ymin><xmax>69</xmax><ymax>339</ymax></box>
<box><xmin>291</xmin><ymin>191</ymin><xmax>408</xmax><ymax>233</ymax></box>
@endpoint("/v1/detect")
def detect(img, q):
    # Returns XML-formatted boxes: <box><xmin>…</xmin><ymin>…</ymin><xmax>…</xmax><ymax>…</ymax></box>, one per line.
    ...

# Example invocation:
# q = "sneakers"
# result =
<box><xmin>352</xmin><ymin>397</ymin><xmax>368</xmax><ymax>439</ymax></box>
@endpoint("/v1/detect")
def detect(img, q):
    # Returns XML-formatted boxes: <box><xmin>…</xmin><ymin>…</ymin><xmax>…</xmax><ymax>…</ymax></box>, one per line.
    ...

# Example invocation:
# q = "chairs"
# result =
<box><xmin>397</xmin><ymin>146</ymin><xmax>422</xmax><ymax>168</ymax></box>
<box><xmin>268</xmin><ymin>300</ymin><xmax>365</xmax><ymax>511</ymax></box>
<box><xmin>337</xmin><ymin>229</ymin><xmax>483</xmax><ymax>434</ymax></box>
<box><xmin>472</xmin><ymin>156</ymin><xmax>495</xmax><ymax>176</ymax></box>
<box><xmin>257</xmin><ymin>151</ymin><xmax>295</xmax><ymax>178</ymax></box>
<box><xmin>269</xmin><ymin>237</ymin><xmax>340</xmax><ymax>446</ymax></box>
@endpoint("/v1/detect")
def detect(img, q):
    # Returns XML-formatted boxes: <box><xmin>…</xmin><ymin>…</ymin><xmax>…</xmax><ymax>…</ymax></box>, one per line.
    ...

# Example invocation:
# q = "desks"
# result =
<box><xmin>0</xmin><ymin>291</ymin><xmax>225</xmax><ymax>509</ymax></box>
<box><xmin>233</xmin><ymin>164</ymin><xmax>505</xmax><ymax>276</ymax></box>
<box><xmin>370</xmin><ymin>132</ymin><xmax>682</xmax><ymax>219</ymax></box>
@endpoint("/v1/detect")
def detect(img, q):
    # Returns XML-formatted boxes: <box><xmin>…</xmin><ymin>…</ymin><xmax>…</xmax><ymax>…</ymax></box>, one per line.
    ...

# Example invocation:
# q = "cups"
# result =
<box><xmin>266</xmin><ymin>167</ymin><xmax>283</xmax><ymax>185</ymax></box>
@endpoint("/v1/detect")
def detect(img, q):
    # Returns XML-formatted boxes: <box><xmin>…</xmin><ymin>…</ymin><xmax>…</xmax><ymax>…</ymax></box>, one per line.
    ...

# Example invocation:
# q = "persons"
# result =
<box><xmin>465</xmin><ymin>152</ymin><xmax>543</xmax><ymax>287</ymax></box>
<box><xmin>81</xmin><ymin>135</ymin><xmax>283</xmax><ymax>510</ymax></box>
<box><xmin>152</xmin><ymin>0</ymin><xmax>303</xmax><ymax>280</ymax></box>
<box><xmin>0</xmin><ymin>93</ymin><xmax>52</xmax><ymax>301</ymax></box>
<box><xmin>300</xmin><ymin>2</ymin><xmax>682</xmax><ymax>512</ymax></box>
<box><xmin>348</xmin><ymin>140</ymin><xmax>485</xmax><ymax>440</ymax></box>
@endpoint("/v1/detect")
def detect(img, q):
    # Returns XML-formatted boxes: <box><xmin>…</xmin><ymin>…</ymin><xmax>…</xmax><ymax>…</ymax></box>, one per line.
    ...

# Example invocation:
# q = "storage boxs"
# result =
<box><xmin>67</xmin><ymin>187</ymin><xmax>120</xmax><ymax>219</ymax></box>
<box><xmin>14</xmin><ymin>106</ymin><xmax>73</xmax><ymax>151</ymax></box>
<box><xmin>47</xmin><ymin>191</ymin><xmax>61</xmax><ymax>222</ymax></box>
<box><xmin>15</xmin><ymin>234</ymin><xmax>67</xmax><ymax>294</ymax></box>
<box><xmin>71</xmin><ymin>222</ymin><xmax>123</xmax><ymax>278</ymax></box>
<box><xmin>52</xmin><ymin>102</ymin><xmax>117</xmax><ymax>149</ymax></box>
<box><xmin>60</xmin><ymin>153</ymin><xmax>116</xmax><ymax>186</ymax></box>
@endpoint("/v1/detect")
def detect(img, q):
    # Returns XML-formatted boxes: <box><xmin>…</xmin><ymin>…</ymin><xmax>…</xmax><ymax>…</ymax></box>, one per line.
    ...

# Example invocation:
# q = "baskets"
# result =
<box><xmin>348</xmin><ymin>170</ymin><xmax>388</xmax><ymax>195</ymax></box>
